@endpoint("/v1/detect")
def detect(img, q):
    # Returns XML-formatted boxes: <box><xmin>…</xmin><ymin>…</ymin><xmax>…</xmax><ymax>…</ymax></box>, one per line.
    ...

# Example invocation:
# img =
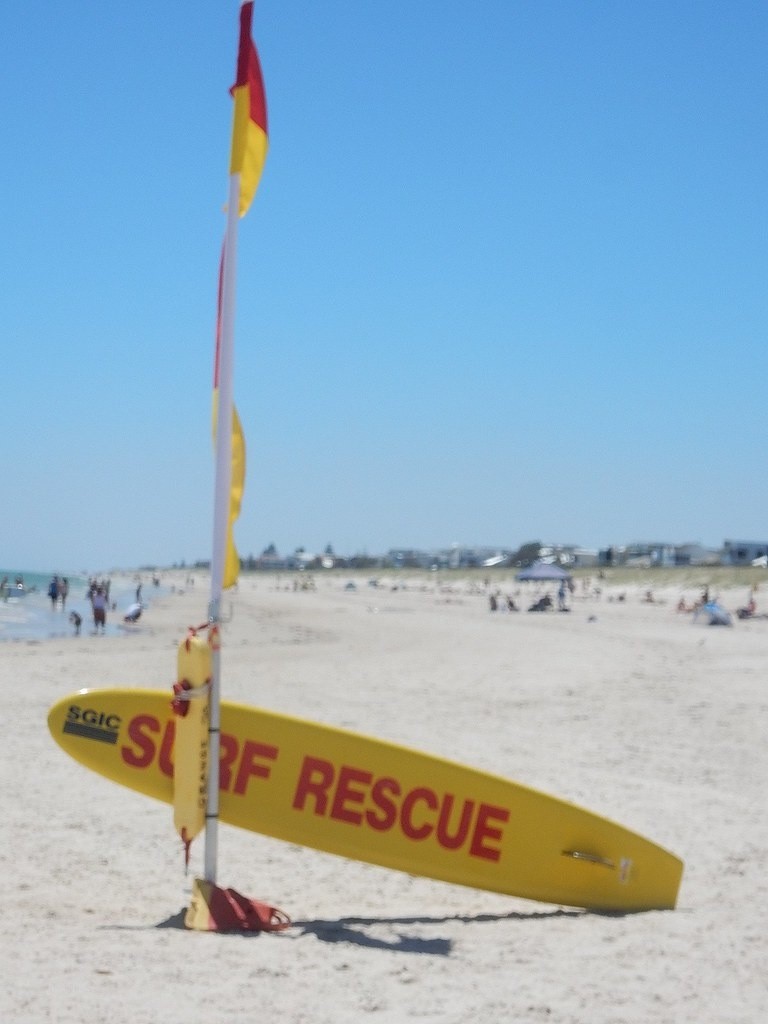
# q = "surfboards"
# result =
<box><xmin>43</xmin><ymin>688</ymin><xmax>684</xmax><ymax>917</ymax></box>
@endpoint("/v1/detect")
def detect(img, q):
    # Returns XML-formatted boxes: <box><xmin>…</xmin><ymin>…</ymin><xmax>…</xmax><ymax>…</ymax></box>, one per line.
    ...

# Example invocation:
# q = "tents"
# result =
<box><xmin>516</xmin><ymin>564</ymin><xmax>574</xmax><ymax>582</ymax></box>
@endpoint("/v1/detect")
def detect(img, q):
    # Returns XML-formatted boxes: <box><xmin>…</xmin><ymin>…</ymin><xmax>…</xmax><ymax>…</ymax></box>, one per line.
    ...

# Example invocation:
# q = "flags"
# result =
<box><xmin>228</xmin><ymin>0</ymin><xmax>269</xmax><ymax>219</ymax></box>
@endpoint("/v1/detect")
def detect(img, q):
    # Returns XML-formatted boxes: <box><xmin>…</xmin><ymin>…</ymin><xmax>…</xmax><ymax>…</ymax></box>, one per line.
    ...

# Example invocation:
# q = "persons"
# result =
<box><xmin>0</xmin><ymin>570</ymin><xmax>759</xmax><ymax>634</ymax></box>
